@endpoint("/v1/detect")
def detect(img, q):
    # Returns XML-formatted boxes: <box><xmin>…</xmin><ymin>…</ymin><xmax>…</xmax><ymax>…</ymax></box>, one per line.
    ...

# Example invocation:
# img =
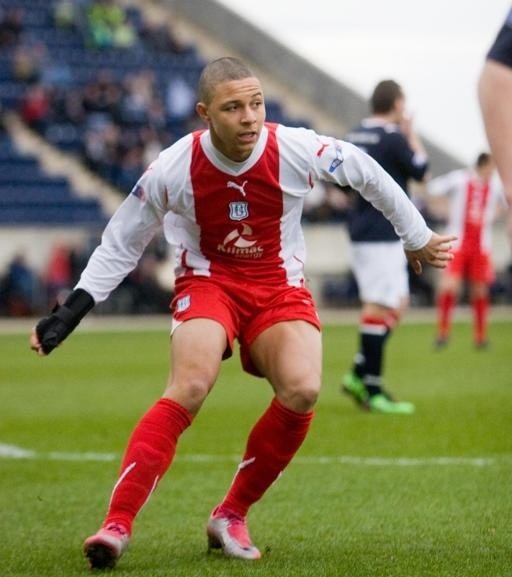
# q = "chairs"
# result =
<box><xmin>0</xmin><ymin>0</ymin><xmax>310</xmax><ymax>226</ymax></box>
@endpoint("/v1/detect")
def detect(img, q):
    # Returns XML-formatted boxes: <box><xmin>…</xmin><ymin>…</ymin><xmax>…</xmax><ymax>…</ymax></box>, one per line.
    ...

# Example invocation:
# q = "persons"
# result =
<box><xmin>334</xmin><ymin>78</ymin><xmax>432</xmax><ymax>409</ymax></box>
<box><xmin>2</xmin><ymin>5</ymin><xmax>210</xmax><ymax>202</ymax></box>
<box><xmin>429</xmin><ymin>153</ymin><xmax>508</xmax><ymax>352</ymax></box>
<box><xmin>1</xmin><ymin>231</ymin><xmax>176</xmax><ymax>317</ymax></box>
<box><xmin>477</xmin><ymin>5</ymin><xmax>511</xmax><ymax>208</ymax></box>
<box><xmin>29</xmin><ymin>55</ymin><xmax>460</xmax><ymax>568</ymax></box>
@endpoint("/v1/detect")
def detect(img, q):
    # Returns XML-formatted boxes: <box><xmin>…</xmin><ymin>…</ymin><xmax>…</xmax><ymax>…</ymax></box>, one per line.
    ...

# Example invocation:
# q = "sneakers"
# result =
<box><xmin>208</xmin><ymin>505</ymin><xmax>261</xmax><ymax>559</ymax></box>
<box><xmin>343</xmin><ymin>374</ymin><xmax>415</xmax><ymax>413</ymax></box>
<box><xmin>84</xmin><ymin>522</ymin><xmax>128</xmax><ymax>569</ymax></box>
